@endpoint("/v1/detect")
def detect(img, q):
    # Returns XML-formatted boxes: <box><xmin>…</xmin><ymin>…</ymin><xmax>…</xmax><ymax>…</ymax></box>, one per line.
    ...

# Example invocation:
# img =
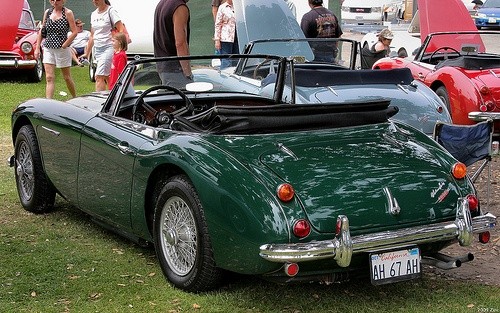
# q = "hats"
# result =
<box><xmin>75</xmin><ymin>19</ymin><xmax>85</xmax><ymax>26</ymax></box>
<box><xmin>379</xmin><ymin>28</ymin><xmax>394</xmax><ymax>39</ymax></box>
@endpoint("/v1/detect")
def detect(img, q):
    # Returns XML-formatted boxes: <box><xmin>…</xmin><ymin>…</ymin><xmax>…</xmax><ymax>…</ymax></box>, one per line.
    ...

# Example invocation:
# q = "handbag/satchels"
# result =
<box><xmin>89</xmin><ymin>63</ymin><xmax>97</xmax><ymax>82</ymax></box>
<box><xmin>111</xmin><ymin>23</ymin><xmax>132</xmax><ymax>45</ymax></box>
<box><xmin>41</xmin><ymin>26</ymin><xmax>46</xmax><ymax>38</ymax></box>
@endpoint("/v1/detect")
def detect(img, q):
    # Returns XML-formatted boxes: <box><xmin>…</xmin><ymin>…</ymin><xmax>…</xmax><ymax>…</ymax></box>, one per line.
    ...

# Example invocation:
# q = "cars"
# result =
<box><xmin>0</xmin><ymin>0</ymin><xmax>45</xmax><ymax>83</ymax></box>
<box><xmin>6</xmin><ymin>52</ymin><xmax>498</xmax><ymax>293</ymax></box>
<box><xmin>89</xmin><ymin>0</ymin><xmax>162</xmax><ymax>81</ymax></box>
<box><xmin>188</xmin><ymin>0</ymin><xmax>454</xmax><ymax>144</ymax></box>
<box><xmin>370</xmin><ymin>0</ymin><xmax>500</xmax><ymax>128</ymax></box>
<box><xmin>474</xmin><ymin>0</ymin><xmax>500</xmax><ymax>29</ymax></box>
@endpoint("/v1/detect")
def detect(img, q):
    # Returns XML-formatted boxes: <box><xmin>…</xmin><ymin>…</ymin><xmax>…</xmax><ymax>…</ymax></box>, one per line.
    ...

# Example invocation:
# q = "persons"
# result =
<box><xmin>211</xmin><ymin>0</ymin><xmax>227</xmax><ymax>26</ymax></box>
<box><xmin>67</xmin><ymin>18</ymin><xmax>95</xmax><ymax>67</ymax></box>
<box><xmin>86</xmin><ymin>1</ymin><xmax>130</xmax><ymax>92</ymax></box>
<box><xmin>300</xmin><ymin>0</ymin><xmax>343</xmax><ymax>64</ymax></box>
<box><xmin>152</xmin><ymin>0</ymin><xmax>196</xmax><ymax>92</ymax></box>
<box><xmin>214</xmin><ymin>0</ymin><xmax>241</xmax><ymax>71</ymax></box>
<box><xmin>106</xmin><ymin>32</ymin><xmax>129</xmax><ymax>89</ymax></box>
<box><xmin>35</xmin><ymin>0</ymin><xmax>81</xmax><ymax>101</ymax></box>
<box><xmin>368</xmin><ymin>28</ymin><xmax>394</xmax><ymax>70</ymax></box>
